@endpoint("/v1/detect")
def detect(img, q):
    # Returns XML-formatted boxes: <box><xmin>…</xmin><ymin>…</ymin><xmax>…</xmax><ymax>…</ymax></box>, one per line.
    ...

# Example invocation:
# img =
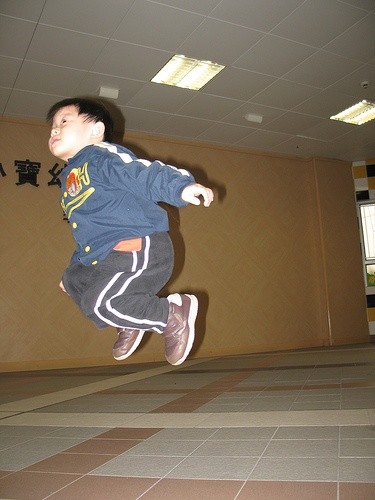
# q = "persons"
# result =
<box><xmin>45</xmin><ymin>97</ymin><xmax>214</xmax><ymax>365</ymax></box>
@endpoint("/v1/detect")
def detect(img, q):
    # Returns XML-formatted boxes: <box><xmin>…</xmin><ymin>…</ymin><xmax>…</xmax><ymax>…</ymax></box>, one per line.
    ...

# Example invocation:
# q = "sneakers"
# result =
<box><xmin>162</xmin><ymin>292</ymin><xmax>199</xmax><ymax>366</ymax></box>
<box><xmin>111</xmin><ymin>325</ymin><xmax>146</xmax><ymax>361</ymax></box>
